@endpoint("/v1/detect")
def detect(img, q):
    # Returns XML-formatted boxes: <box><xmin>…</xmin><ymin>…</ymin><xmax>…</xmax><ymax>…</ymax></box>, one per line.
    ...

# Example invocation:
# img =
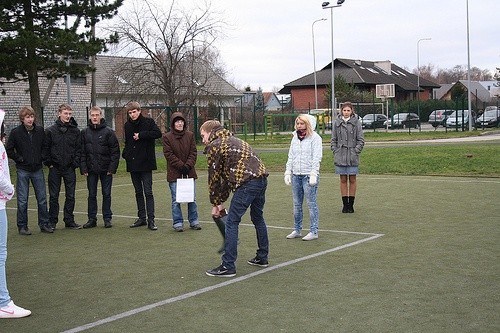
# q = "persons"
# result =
<box><xmin>285</xmin><ymin>114</ymin><xmax>322</xmax><ymax>240</ymax></box>
<box><xmin>80</xmin><ymin>106</ymin><xmax>120</xmax><ymax>228</ymax></box>
<box><xmin>330</xmin><ymin>103</ymin><xmax>365</xmax><ymax>213</ymax></box>
<box><xmin>122</xmin><ymin>102</ymin><xmax>161</xmax><ymax>230</ymax></box>
<box><xmin>163</xmin><ymin>112</ymin><xmax>202</xmax><ymax>232</ymax></box>
<box><xmin>200</xmin><ymin>120</ymin><xmax>269</xmax><ymax>277</ymax></box>
<box><xmin>0</xmin><ymin>109</ymin><xmax>32</xmax><ymax>318</ymax></box>
<box><xmin>43</xmin><ymin>104</ymin><xmax>81</xmax><ymax>230</ymax></box>
<box><xmin>6</xmin><ymin>106</ymin><xmax>55</xmax><ymax>234</ymax></box>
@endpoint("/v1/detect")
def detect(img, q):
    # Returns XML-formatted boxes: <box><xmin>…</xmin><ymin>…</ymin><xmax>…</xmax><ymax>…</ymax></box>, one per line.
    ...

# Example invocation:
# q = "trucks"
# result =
<box><xmin>306</xmin><ymin>107</ymin><xmax>342</xmax><ymax>128</ymax></box>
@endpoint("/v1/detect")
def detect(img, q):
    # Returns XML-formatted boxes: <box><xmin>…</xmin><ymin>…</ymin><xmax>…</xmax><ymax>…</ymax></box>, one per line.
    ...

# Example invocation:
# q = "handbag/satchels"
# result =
<box><xmin>176</xmin><ymin>173</ymin><xmax>195</xmax><ymax>203</ymax></box>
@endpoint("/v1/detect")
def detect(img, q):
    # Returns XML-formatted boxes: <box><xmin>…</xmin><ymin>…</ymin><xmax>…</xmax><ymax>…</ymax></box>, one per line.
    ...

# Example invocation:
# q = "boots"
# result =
<box><xmin>341</xmin><ymin>195</ymin><xmax>349</xmax><ymax>213</ymax></box>
<box><xmin>348</xmin><ymin>196</ymin><xmax>355</xmax><ymax>213</ymax></box>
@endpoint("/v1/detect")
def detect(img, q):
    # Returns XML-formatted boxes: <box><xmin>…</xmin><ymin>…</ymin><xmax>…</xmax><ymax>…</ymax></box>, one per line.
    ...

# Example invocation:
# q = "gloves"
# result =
<box><xmin>284</xmin><ymin>175</ymin><xmax>291</xmax><ymax>186</ymax></box>
<box><xmin>310</xmin><ymin>176</ymin><xmax>317</xmax><ymax>186</ymax></box>
<box><xmin>180</xmin><ymin>165</ymin><xmax>189</xmax><ymax>174</ymax></box>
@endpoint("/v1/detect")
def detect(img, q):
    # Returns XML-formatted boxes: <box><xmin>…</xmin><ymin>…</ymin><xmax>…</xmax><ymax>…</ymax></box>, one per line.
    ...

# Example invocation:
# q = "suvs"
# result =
<box><xmin>428</xmin><ymin>108</ymin><xmax>455</xmax><ymax>128</ymax></box>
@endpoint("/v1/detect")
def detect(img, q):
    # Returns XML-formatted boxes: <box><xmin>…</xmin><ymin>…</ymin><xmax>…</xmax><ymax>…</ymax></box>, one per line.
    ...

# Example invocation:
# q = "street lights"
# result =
<box><xmin>321</xmin><ymin>0</ymin><xmax>346</xmax><ymax>139</ymax></box>
<box><xmin>416</xmin><ymin>38</ymin><xmax>432</xmax><ymax>116</ymax></box>
<box><xmin>312</xmin><ymin>18</ymin><xmax>328</xmax><ymax>109</ymax></box>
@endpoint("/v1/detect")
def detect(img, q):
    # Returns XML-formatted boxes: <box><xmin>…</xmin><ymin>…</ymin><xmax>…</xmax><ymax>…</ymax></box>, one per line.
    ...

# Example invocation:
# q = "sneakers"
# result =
<box><xmin>286</xmin><ymin>230</ymin><xmax>302</xmax><ymax>238</ymax></box>
<box><xmin>302</xmin><ymin>232</ymin><xmax>318</xmax><ymax>241</ymax></box>
<box><xmin>148</xmin><ymin>223</ymin><xmax>158</xmax><ymax>230</ymax></box>
<box><xmin>174</xmin><ymin>227</ymin><xmax>184</xmax><ymax>231</ymax></box>
<box><xmin>205</xmin><ymin>263</ymin><xmax>236</xmax><ymax>277</ymax></box>
<box><xmin>18</xmin><ymin>226</ymin><xmax>32</xmax><ymax>235</ymax></box>
<box><xmin>128</xmin><ymin>219</ymin><xmax>146</xmax><ymax>228</ymax></box>
<box><xmin>192</xmin><ymin>224</ymin><xmax>201</xmax><ymax>230</ymax></box>
<box><xmin>0</xmin><ymin>300</ymin><xmax>31</xmax><ymax>318</ymax></box>
<box><xmin>248</xmin><ymin>255</ymin><xmax>269</xmax><ymax>267</ymax></box>
<box><xmin>65</xmin><ymin>222</ymin><xmax>81</xmax><ymax>229</ymax></box>
<box><xmin>40</xmin><ymin>224</ymin><xmax>54</xmax><ymax>233</ymax></box>
<box><xmin>105</xmin><ymin>219</ymin><xmax>112</xmax><ymax>228</ymax></box>
<box><xmin>83</xmin><ymin>220</ymin><xmax>97</xmax><ymax>228</ymax></box>
<box><xmin>49</xmin><ymin>221</ymin><xmax>56</xmax><ymax>230</ymax></box>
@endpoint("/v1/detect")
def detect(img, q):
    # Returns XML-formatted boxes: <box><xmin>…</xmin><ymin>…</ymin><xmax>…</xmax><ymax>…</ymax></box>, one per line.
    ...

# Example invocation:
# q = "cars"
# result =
<box><xmin>385</xmin><ymin>113</ymin><xmax>422</xmax><ymax>130</ymax></box>
<box><xmin>445</xmin><ymin>108</ymin><xmax>477</xmax><ymax>128</ymax></box>
<box><xmin>361</xmin><ymin>113</ymin><xmax>391</xmax><ymax>129</ymax></box>
<box><xmin>475</xmin><ymin>109</ymin><xmax>500</xmax><ymax>129</ymax></box>
